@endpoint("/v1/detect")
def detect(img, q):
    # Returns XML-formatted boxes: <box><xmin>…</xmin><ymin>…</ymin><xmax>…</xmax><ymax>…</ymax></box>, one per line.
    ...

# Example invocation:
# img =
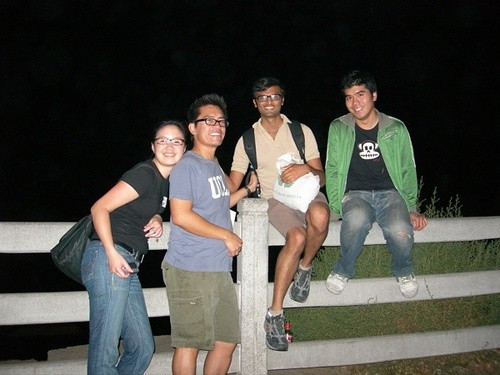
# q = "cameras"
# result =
<box><xmin>128</xmin><ymin>262</ymin><xmax>139</xmax><ymax>273</ymax></box>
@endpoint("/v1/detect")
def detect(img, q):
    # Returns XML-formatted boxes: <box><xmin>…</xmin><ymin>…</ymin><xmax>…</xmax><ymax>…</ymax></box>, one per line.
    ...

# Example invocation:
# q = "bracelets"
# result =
<box><xmin>244</xmin><ymin>183</ymin><xmax>251</xmax><ymax>196</ymax></box>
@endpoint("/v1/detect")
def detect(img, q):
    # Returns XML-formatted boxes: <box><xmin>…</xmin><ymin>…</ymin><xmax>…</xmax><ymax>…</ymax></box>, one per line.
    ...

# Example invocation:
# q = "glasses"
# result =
<box><xmin>195</xmin><ymin>118</ymin><xmax>230</xmax><ymax>127</ymax></box>
<box><xmin>256</xmin><ymin>94</ymin><xmax>281</xmax><ymax>102</ymax></box>
<box><xmin>154</xmin><ymin>137</ymin><xmax>184</xmax><ymax>146</ymax></box>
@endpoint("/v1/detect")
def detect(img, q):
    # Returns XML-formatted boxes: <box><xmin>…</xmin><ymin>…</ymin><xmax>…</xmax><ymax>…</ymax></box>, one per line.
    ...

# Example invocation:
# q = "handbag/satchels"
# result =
<box><xmin>52</xmin><ymin>214</ymin><xmax>95</xmax><ymax>284</ymax></box>
<box><xmin>273</xmin><ymin>152</ymin><xmax>321</xmax><ymax>214</ymax></box>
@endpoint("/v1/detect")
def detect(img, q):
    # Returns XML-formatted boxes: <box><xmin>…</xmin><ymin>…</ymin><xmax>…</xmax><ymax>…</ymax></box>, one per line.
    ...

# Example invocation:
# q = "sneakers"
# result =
<box><xmin>290</xmin><ymin>264</ymin><xmax>311</xmax><ymax>302</ymax></box>
<box><xmin>395</xmin><ymin>271</ymin><xmax>417</xmax><ymax>298</ymax></box>
<box><xmin>264</xmin><ymin>307</ymin><xmax>288</xmax><ymax>351</ymax></box>
<box><xmin>326</xmin><ymin>270</ymin><xmax>348</xmax><ymax>294</ymax></box>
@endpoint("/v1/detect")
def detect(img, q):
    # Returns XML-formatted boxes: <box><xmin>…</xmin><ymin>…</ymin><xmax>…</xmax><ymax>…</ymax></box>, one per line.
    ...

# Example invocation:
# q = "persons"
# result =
<box><xmin>325</xmin><ymin>70</ymin><xmax>428</xmax><ymax>298</ymax></box>
<box><xmin>161</xmin><ymin>93</ymin><xmax>259</xmax><ymax>375</ymax></box>
<box><xmin>81</xmin><ymin>121</ymin><xmax>188</xmax><ymax>375</ymax></box>
<box><xmin>224</xmin><ymin>77</ymin><xmax>331</xmax><ymax>352</ymax></box>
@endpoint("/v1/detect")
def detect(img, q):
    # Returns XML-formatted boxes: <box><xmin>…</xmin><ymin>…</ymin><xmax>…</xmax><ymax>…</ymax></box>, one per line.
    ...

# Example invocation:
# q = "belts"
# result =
<box><xmin>92</xmin><ymin>238</ymin><xmax>144</xmax><ymax>264</ymax></box>
